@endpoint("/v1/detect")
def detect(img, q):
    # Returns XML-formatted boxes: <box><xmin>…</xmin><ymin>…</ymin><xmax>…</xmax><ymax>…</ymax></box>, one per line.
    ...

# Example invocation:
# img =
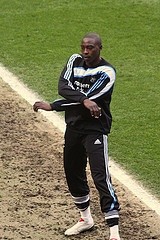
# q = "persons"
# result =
<box><xmin>33</xmin><ymin>32</ymin><xmax>120</xmax><ymax>240</ymax></box>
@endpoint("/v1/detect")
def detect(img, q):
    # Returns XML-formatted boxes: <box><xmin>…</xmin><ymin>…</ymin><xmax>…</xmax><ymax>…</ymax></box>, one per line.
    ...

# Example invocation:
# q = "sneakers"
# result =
<box><xmin>109</xmin><ymin>235</ymin><xmax>120</xmax><ymax>240</ymax></box>
<box><xmin>65</xmin><ymin>218</ymin><xmax>94</xmax><ymax>236</ymax></box>
